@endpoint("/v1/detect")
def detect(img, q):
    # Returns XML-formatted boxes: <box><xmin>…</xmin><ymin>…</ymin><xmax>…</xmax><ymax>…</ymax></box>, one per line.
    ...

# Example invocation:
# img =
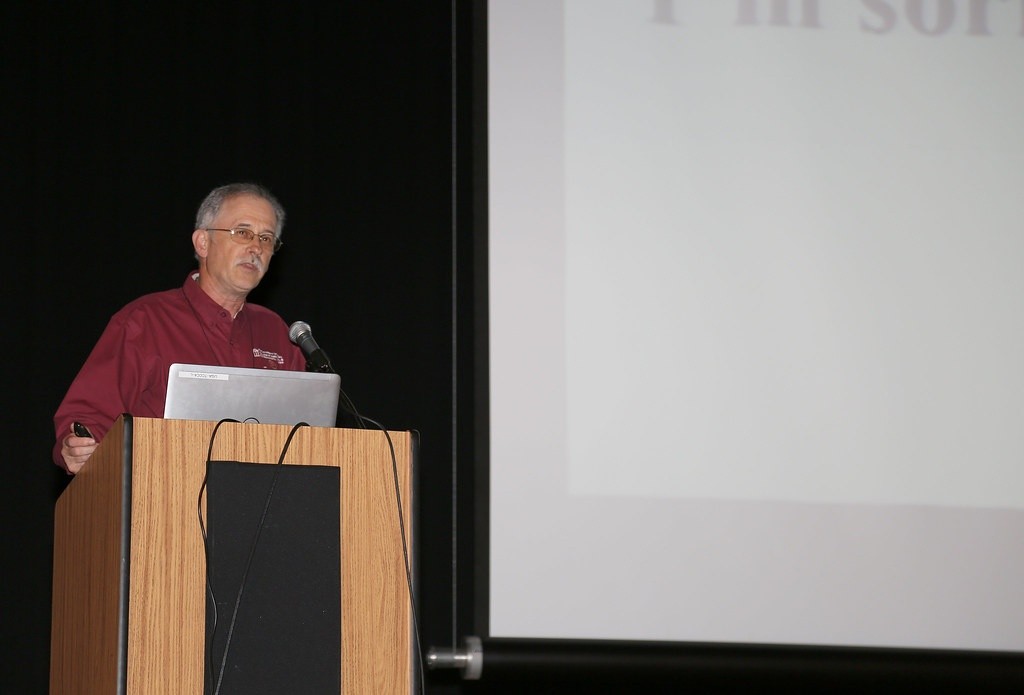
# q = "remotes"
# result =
<box><xmin>74</xmin><ymin>422</ymin><xmax>93</xmax><ymax>438</ymax></box>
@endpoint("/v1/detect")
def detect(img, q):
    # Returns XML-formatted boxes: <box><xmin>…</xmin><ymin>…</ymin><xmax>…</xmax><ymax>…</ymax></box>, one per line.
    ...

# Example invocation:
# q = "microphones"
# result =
<box><xmin>289</xmin><ymin>321</ymin><xmax>336</xmax><ymax>375</ymax></box>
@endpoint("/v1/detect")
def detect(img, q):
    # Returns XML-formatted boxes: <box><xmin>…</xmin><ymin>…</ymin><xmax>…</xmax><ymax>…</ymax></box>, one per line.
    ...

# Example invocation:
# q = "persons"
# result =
<box><xmin>52</xmin><ymin>183</ymin><xmax>305</xmax><ymax>476</ymax></box>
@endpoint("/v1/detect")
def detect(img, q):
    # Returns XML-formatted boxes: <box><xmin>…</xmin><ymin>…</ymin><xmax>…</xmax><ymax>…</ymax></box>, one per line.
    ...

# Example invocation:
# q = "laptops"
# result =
<box><xmin>163</xmin><ymin>363</ymin><xmax>341</xmax><ymax>429</ymax></box>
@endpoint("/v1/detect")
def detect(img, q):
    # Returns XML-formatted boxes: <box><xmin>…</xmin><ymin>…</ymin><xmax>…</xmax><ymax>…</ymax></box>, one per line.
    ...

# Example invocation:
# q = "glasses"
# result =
<box><xmin>205</xmin><ymin>227</ymin><xmax>283</xmax><ymax>252</ymax></box>
